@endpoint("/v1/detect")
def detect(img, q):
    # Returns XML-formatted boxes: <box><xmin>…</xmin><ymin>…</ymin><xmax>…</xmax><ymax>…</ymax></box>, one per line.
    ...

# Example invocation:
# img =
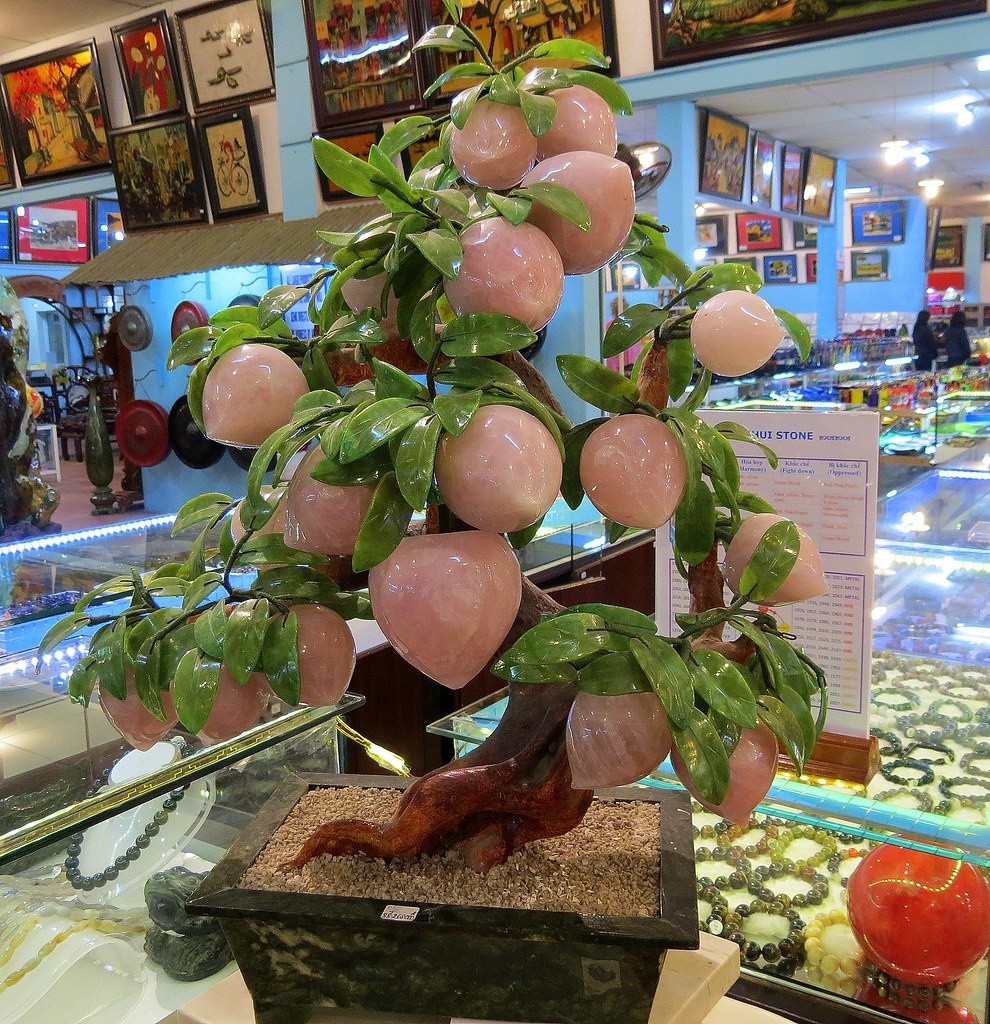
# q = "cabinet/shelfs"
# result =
<box><xmin>35</xmin><ymin>425</ymin><xmax>62</xmax><ymax>483</ymax></box>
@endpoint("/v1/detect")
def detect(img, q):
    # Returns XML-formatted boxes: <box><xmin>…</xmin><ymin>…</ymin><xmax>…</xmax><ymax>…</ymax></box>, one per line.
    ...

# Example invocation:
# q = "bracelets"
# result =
<box><xmin>666</xmin><ymin>593</ymin><xmax>990</xmax><ymax>1013</ymax></box>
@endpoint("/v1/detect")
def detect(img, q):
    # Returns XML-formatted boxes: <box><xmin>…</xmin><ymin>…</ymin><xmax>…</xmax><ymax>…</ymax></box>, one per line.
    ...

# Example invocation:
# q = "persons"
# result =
<box><xmin>945</xmin><ymin>311</ymin><xmax>971</xmax><ymax>368</ymax></box>
<box><xmin>912</xmin><ymin>311</ymin><xmax>938</xmax><ymax>371</ymax></box>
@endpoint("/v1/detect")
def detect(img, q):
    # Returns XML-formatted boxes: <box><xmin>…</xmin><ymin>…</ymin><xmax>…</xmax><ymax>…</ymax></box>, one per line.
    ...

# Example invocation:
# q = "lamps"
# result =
<box><xmin>880</xmin><ymin>86</ymin><xmax>909</xmax><ymax>164</ymax></box>
<box><xmin>918</xmin><ymin>62</ymin><xmax>944</xmax><ymax>199</ymax></box>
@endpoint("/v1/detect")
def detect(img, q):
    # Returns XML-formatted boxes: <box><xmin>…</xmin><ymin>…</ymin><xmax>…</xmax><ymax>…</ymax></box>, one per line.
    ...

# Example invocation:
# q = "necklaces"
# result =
<box><xmin>65</xmin><ymin>735</ymin><xmax>191</xmax><ymax>891</ymax></box>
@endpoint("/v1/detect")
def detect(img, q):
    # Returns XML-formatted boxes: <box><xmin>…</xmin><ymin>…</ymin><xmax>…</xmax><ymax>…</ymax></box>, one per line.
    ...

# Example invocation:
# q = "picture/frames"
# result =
<box><xmin>302</xmin><ymin>0</ymin><xmax>621</xmax><ymax>201</ymax></box>
<box><xmin>0</xmin><ymin>0</ymin><xmax>277</xmax><ymax>264</ymax></box>
<box><xmin>983</xmin><ymin>223</ymin><xmax>990</xmax><ymax>261</ymax></box>
<box><xmin>695</xmin><ymin>105</ymin><xmax>905</xmax><ymax>284</ymax></box>
<box><xmin>924</xmin><ymin>225</ymin><xmax>964</xmax><ymax>268</ymax></box>
<box><xmin>650</xmin><ymin>1</ymin><xmax>986</xmax><ymax>69</ymax></box>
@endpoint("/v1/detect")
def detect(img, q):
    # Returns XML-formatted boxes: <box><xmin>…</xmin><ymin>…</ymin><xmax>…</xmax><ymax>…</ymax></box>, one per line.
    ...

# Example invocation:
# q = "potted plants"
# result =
<box><xmin>33</xmin><ymin>0</ymin><xmax>830</xmax><ymax>1024</ymax></box>
